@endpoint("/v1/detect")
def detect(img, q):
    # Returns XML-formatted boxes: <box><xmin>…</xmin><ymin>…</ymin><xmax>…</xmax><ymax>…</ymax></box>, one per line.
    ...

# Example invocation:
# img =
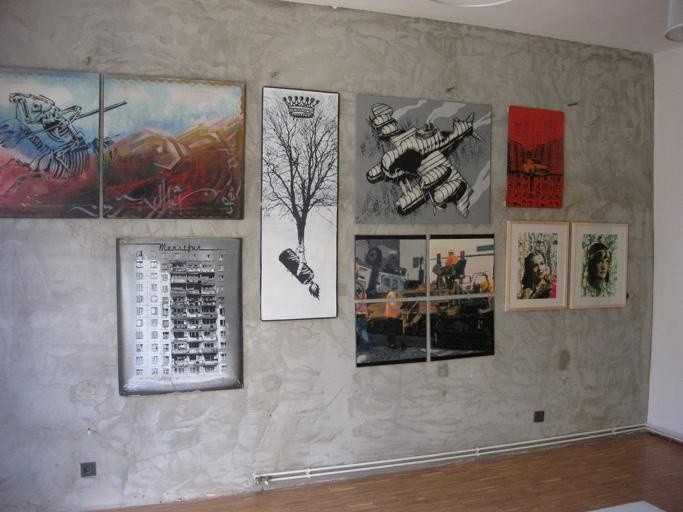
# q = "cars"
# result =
<box><xmin>519</xmin><ymin>157</ymin><xmax>549</xmax><ymax>177</ymax></box>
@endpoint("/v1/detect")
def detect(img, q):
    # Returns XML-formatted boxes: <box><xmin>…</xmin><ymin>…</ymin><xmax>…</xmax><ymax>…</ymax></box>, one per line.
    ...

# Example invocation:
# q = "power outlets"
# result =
<box><xmin>532</xmin><ymin>410</ymin><xmax>544</xmax><ymax>423</ymax></box>
<box><xmin>79</xmin><ymin>461</ymin><xmax>96</xmax><ymax>478</ymax></box>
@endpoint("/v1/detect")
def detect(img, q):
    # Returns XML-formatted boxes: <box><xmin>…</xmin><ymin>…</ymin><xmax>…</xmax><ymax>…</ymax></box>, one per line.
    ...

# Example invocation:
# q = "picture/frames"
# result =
<box><xmin>504</xmin><ymin>218</ymin><xmax>629</xmax><ymax>313</ymax></box>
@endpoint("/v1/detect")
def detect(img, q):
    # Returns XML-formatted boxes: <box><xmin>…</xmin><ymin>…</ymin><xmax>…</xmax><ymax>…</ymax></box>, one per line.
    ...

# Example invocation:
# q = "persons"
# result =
<box><xmin>384</xmin><ymin>285</ymin><xmax>402</xmax><ymax>347</ymax></box>
<box><xmin>446</xmin><ymin>250</ymin><xmax>466</xmax><ymax>290</ymax></box>
<box><xmin>442</xmin><ymin>251</ymin><xmax>457</xmax><ymax>271</ymax></box>
<box><xmin>520</xmin><ymin>248</ymin><xmax>558</xmax><ymax>300</ymax></box>
<box><xmin>583</xmin><ymin>242</ymin><xmax>613</xmax><ymax>298</ymax></box>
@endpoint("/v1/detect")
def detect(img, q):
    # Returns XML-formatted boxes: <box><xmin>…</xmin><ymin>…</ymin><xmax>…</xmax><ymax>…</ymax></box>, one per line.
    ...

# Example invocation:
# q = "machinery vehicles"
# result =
<box><xmin>357</xmin><ymin>249</ymin><xmax>491</xmax><ymax>343</ymax></box>
